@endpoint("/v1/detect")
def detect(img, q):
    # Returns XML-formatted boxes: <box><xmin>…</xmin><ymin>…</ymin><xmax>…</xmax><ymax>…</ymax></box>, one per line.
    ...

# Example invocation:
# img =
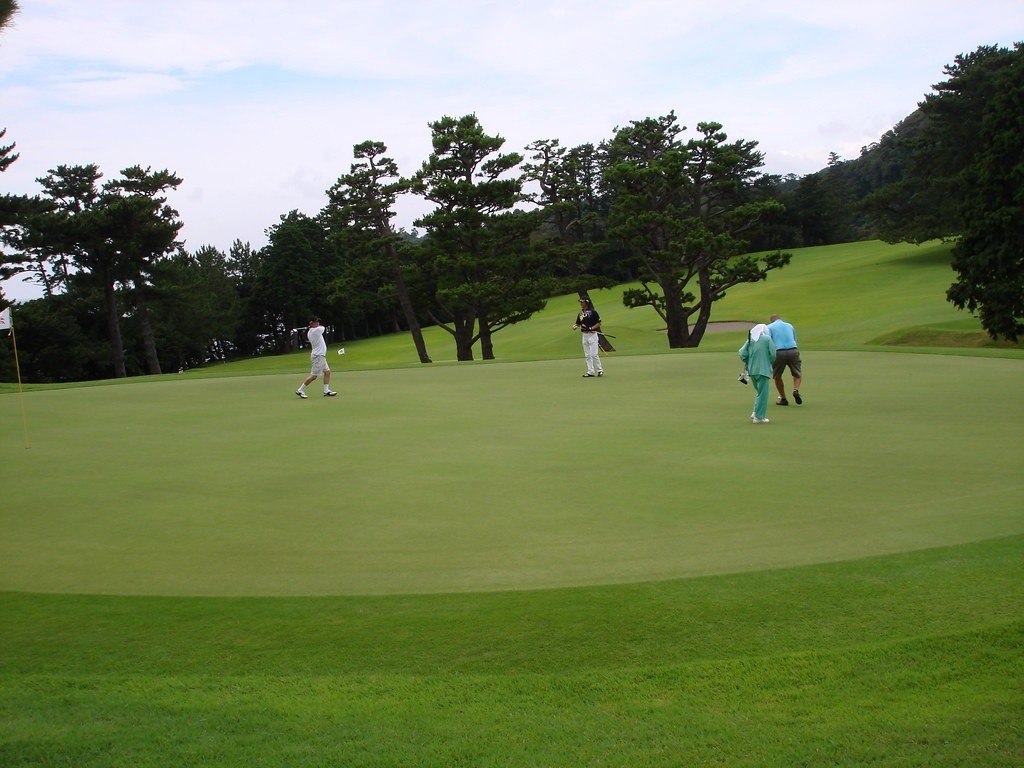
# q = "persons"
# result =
<box><xmin>766</xmin><ymin>314</ymin><xmax>802</xmax><ymax>405</ymax></box>
<box><xmin>739</xmin><ymin>324</ymin><xmax>777</xmax><ymax>424</ymax></box>
<box><xmin>572</xmin><ymin>296</ymin><xmax>604</xmax><ymax>377</ymax></box>
<box><xmin>296</xmin><ymin>315</ymin><xmax>337</xmax><ymax>399</ymax></box>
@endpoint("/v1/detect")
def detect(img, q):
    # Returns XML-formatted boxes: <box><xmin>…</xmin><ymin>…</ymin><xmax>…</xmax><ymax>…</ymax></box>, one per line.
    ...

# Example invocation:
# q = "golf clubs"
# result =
<box><xmin>577</xmin><ymin>326</ymin><xmax>616</xmax><ymax>339</ymax></box>
<box><xmin>292</xmin><ymin>327</ymin><xmax>310</xmax><ymax>332</ymax></box>
<box><xmin>738</xmin><ymin>328</ymin><xmax>752</xmax><ymax>386</ymax></box>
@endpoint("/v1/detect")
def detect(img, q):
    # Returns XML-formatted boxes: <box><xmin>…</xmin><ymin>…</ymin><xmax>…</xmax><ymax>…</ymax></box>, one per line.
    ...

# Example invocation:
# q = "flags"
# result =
<box><xmin>0</xmin><ymin>306</ymin><xmax>12</xmax><ymax>329</ymax></box>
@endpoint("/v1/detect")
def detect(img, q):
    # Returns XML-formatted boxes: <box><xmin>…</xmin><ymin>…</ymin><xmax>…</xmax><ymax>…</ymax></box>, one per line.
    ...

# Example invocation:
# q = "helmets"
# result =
<box><xmin>579</xmin><ymin>296</ymin><xmax>592</xmax><ymax>304</ymax></box>
<box><xmin>306</xmin><ymin>315</ymin><xmax>321</xmax><ymax>324</ymax></box>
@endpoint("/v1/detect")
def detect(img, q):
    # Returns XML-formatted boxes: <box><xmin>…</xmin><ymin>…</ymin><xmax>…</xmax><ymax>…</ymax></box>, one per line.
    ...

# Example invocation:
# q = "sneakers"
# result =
<box><xmin>751</xmin><ymin>411</ymin><xmax>770</xmax><ymax>423</ymax></box>
<box><xmin>776</xmin><ymin>399</ymin><xmax>789</xmax><ymax>406</ymax></box>
<box><xmin>598</xmin><ymin>372</ymin><xmax>603</xmax><ymax>376</ymax></box>
<box><xmin>792</xmin><ymin>391</ymin><xmax>803</xmax><ymax>405</ymax></box>
<box><xmin>295</xmin><ymin>390</ymin><xmax>307</xmax><ymax>398</ymax></box>
<box><xmin>324</xmin><ymin>391</ymin><xmax>337</xmax><ymax>396</ymax></box>
<box><xmin>582</xmin><ymin>373</ymin><xmax>596</xmax><ymax>377</ymax></box>
